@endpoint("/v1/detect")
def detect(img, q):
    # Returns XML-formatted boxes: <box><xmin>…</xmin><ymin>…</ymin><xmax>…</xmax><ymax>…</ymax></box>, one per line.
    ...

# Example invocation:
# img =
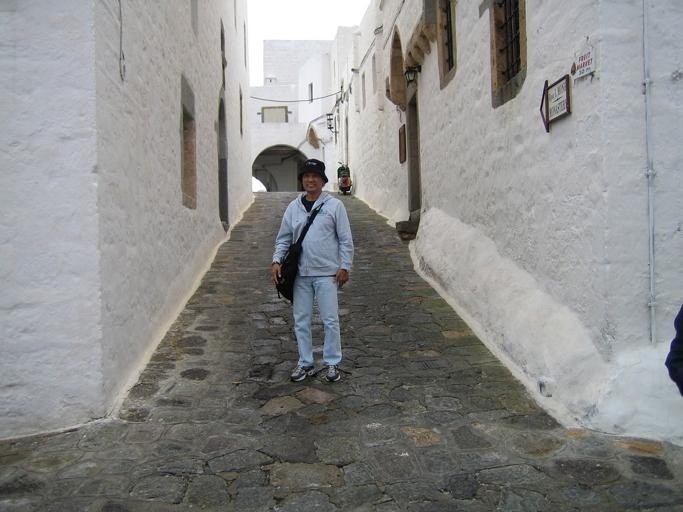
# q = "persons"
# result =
<box><xmin>271</xmin><ymin>159</ymin><xmax>355</xmax><ymax>383</ymax></box>
<box><xmin>664</xmin><ymin>304</ymin><xmax>683</xmax><ymax>395</ymax></box>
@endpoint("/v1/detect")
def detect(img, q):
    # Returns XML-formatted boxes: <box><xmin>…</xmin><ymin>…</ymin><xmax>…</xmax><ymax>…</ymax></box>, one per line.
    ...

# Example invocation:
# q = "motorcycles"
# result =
<box><xmin>336</xmin><ymin>161</ymin><xmax>351</xmax><ymax>195</ymax></box>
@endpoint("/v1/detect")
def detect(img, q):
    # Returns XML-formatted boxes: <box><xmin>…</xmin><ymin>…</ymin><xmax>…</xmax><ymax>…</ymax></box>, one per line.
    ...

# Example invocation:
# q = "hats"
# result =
<box><xmin>298</xmin><ymin>159</ymin><xmax>327</xmax><ymax>183</ymax></box>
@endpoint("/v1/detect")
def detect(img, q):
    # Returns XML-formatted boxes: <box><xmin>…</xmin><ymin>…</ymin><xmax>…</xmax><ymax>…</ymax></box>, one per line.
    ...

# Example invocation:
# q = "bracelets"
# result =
<box><xmin>271</xmin><ymin>262</ymin><xmax>279</xmax><ymax>266</ymax></box>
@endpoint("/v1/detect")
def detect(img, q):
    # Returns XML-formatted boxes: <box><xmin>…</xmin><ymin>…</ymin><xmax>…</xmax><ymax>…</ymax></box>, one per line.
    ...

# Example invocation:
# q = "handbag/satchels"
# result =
<box><xmin>276</xmin><ymin>243</ymin><xmax>298</xmax><ymax>302</ymax></box>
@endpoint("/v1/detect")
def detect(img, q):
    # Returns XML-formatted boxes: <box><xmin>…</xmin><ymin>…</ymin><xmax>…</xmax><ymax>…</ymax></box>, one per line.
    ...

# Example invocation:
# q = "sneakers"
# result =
<box><xmin>291</xmin><ymin>366</ymin><xmax>315</xmax><ymax>381</ymax></box>
<box><xmin>326</xmin><ymin>366</ymin><xmax>340</xmax><ymax>381</ymax></box>
